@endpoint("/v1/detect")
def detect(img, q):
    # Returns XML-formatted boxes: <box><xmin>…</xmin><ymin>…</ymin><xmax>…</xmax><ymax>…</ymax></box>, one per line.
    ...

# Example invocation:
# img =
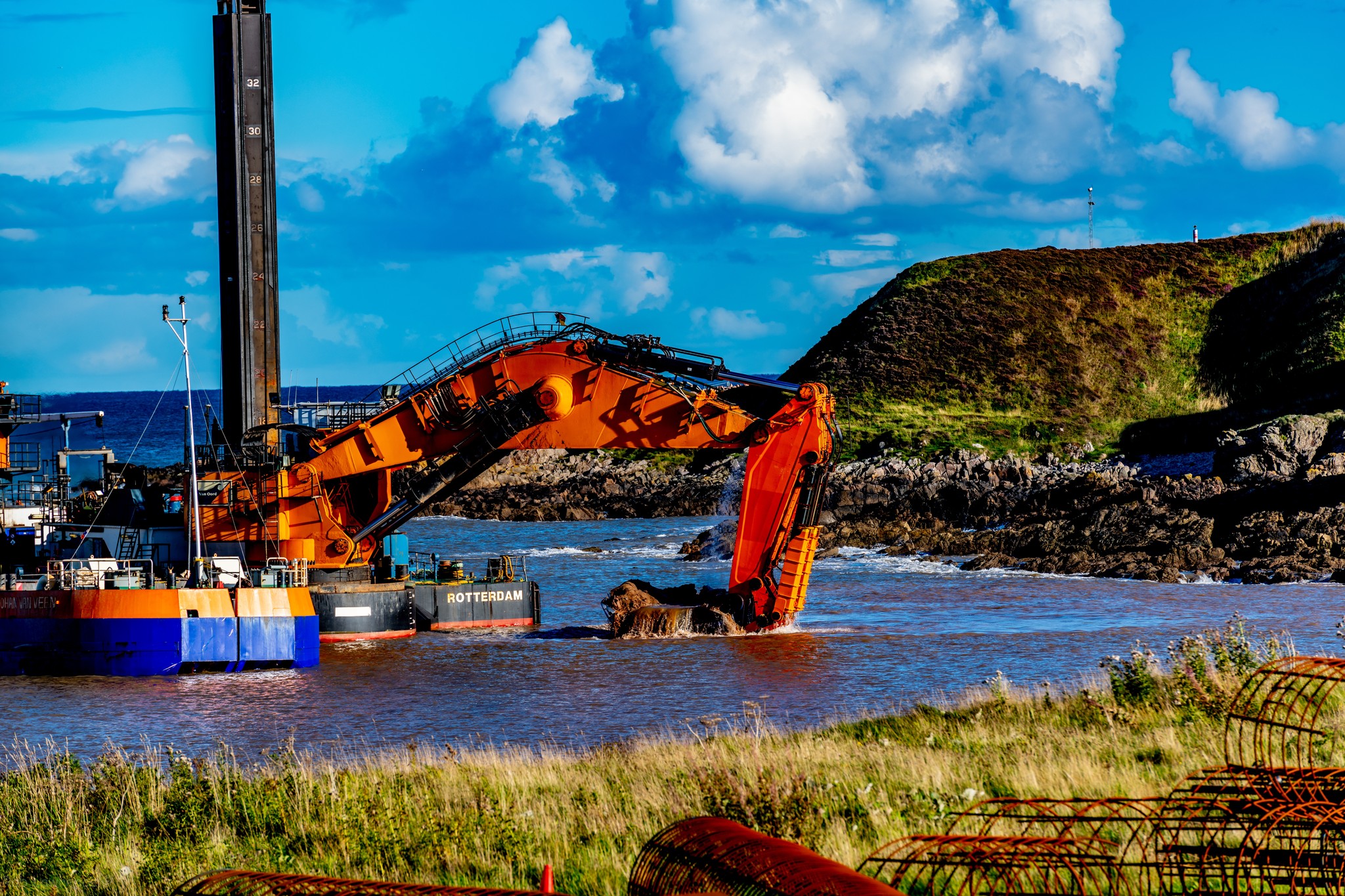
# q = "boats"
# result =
<box><xmin>0</xmin><ymin>381</ymin><xmax>543</xmax><ymax>679</ymax></box>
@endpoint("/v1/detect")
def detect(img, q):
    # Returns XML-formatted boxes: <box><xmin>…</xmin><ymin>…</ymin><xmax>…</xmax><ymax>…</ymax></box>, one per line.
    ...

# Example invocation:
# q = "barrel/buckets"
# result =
<box><xmin>395</xmin><ymin>564</ymin><xmax>408</xmax><ymax>580</ymax></box>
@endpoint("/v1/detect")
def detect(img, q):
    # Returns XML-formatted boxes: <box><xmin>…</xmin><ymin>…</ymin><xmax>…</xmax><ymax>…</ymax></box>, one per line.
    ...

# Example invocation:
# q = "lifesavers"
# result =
<box><xmin>36</xmin><ymin>574</ymin><xmax>57</xmax><ymax>591</ymax></box>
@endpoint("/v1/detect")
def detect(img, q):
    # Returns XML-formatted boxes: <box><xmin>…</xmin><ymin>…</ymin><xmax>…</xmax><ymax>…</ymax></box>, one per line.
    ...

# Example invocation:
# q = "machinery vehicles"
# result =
<box><xmin>182</xmin><ymin>311</ymin><xmax>852</xmax><ymax>640</ymax></box>
